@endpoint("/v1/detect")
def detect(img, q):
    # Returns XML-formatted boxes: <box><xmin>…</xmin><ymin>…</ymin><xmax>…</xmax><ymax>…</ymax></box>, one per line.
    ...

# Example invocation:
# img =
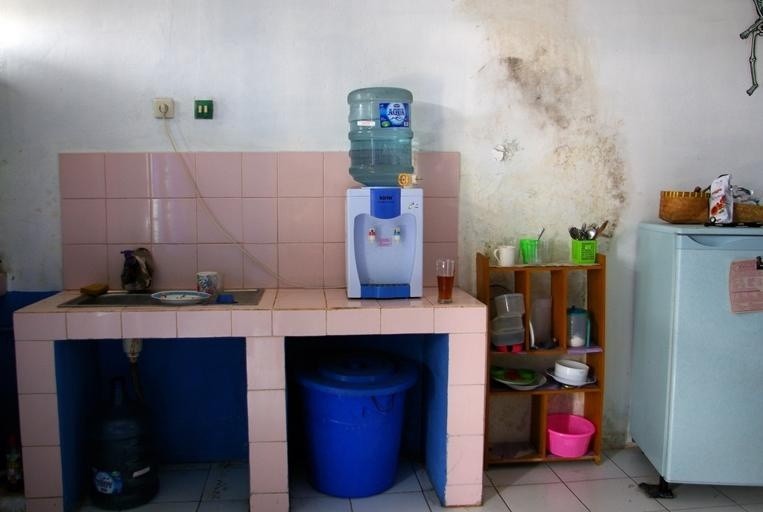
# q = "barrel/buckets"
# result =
<box><xmin>348</xmin><ymin>88</ymin><xmax>414</xmax><ymax>186</ymax></box>
<box><xmin>303</xmin><ymin>359</ymin><xmax>421</xmax><ymax>498</ymax></box>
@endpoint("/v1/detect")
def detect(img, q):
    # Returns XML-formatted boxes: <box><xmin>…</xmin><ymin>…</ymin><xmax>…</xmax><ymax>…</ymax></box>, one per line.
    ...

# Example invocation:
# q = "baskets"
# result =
<box><xmin>657</xmin><ymin>189</ymin><xmax>762</xmax><ymax>226</ymax></box>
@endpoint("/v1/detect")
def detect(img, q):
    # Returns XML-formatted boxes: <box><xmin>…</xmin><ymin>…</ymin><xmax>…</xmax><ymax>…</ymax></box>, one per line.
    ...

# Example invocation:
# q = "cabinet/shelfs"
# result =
<box><xmin>475</xmin><ymin>254</ymin><xmax>606</xmax><ymax>465</ymax></box>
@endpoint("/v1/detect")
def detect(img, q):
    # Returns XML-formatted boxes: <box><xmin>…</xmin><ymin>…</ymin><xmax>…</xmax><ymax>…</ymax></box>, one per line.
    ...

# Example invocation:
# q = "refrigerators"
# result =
<box><xmin>629</xmin><ymin>222</ymin><xmax>762</xmax><ymax>497</ymax></box>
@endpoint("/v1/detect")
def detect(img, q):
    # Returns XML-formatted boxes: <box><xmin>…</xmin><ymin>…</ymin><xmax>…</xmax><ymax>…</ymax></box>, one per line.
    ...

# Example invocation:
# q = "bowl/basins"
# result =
<box><xmin>545</xmin><ymin>413</ymin><xmax>596</xmax><ymax>459</ymax></box>
<box><xmin>555</xmin><ymin>359</ymin><xmax>589</xmax><ymax>381</ymax></box>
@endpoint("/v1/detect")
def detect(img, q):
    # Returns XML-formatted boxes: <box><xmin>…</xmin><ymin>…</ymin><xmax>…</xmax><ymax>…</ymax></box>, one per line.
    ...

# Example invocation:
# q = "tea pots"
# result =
<box><xmin>118</xmin><ymin>246</ymin><xmax>156</xmax><ymax>291</ymax></box>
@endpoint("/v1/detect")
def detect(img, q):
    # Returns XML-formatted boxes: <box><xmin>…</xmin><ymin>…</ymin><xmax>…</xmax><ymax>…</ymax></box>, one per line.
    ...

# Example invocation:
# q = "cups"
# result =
<box><xmin>435</xmin><ymin>259</ymin><xmax>455</xmax><ymax>305</ymax></box>
<box><xmin>197</xmin><ymin>271</ymin><xmax>221</xmax><ymax>295</ymax></box>
<box><xmin>492</xmin><ymin>239</ymin><xmax>539</xmax><ymax>268</ymax></box>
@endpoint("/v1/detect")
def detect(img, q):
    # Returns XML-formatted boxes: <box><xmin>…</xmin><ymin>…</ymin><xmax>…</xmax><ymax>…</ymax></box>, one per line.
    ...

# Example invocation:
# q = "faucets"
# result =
<box><xmin>368</xmin><ymin>228</ymin><xmax>375</xmax><ymax>243</ymax></box>
<box><xmin>393</xmin><ymin>227</ymin><xmax>401</xmax><ymax>245</ymax></box>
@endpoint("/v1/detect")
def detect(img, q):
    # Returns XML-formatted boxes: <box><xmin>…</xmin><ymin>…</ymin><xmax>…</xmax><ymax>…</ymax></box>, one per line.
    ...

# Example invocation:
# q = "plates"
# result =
<box><xmin>491</xmin><ymin>365</ymin><xmax>547</xmax><ymax>391</ymax></box>
<box><xmin>151</xmin><ymin>290</ymin><xmax>213</xmax><ymax>306</ymax></box>
<box><xmin>545</xmin><ymin>367</ymin><xmax>597</xmax><ymax>388</ymax></box>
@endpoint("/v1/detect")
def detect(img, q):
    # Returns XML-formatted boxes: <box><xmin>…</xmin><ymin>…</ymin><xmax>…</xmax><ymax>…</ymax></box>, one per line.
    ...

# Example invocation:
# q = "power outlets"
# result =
<box><xmin>152</xmin><ymin>98</ymin><xmax>174</xmax><ymax>119</ymax></box>
